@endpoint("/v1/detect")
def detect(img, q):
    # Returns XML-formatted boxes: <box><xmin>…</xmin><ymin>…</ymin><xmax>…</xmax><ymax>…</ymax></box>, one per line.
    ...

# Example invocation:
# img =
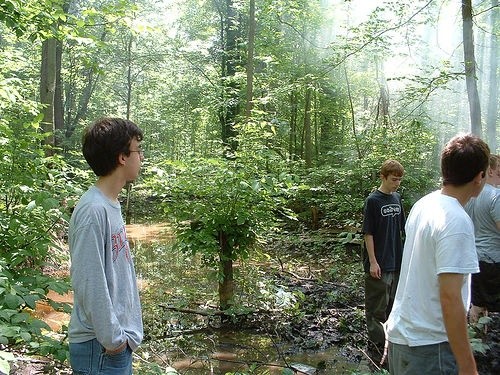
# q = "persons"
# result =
<box><xmin>68</xmin><ymin>117</ymin><xmax>144</xmax><ymax>375</ymax></box>
<box><xmin>462</xmin><ymin>154</ymin><xmax>500</xmax><ymax>334</ymax></box>
<box><xmin>362</xmin><ymin>159</ymin><xmax>407</xmax><ymax>369</ymax></box>
<box><xmin>384</xmin><ymin>134</ymin><xmax>490</xmax><ymax>375</ymax></box>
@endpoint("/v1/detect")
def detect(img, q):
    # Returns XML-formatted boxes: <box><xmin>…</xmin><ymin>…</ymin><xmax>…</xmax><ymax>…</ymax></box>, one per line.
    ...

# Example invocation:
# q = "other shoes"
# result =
<box><xmin>368</xmin><ymin>347</ymin><xmax>387</xmax><ymax>365</ymax></box>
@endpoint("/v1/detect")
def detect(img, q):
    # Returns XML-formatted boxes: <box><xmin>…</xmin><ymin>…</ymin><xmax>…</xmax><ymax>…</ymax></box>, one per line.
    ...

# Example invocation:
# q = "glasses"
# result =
<box><xmin>126</xmin><ymin>150</ymin><xmax>145</xmax><ymax>156</ymax></box>
